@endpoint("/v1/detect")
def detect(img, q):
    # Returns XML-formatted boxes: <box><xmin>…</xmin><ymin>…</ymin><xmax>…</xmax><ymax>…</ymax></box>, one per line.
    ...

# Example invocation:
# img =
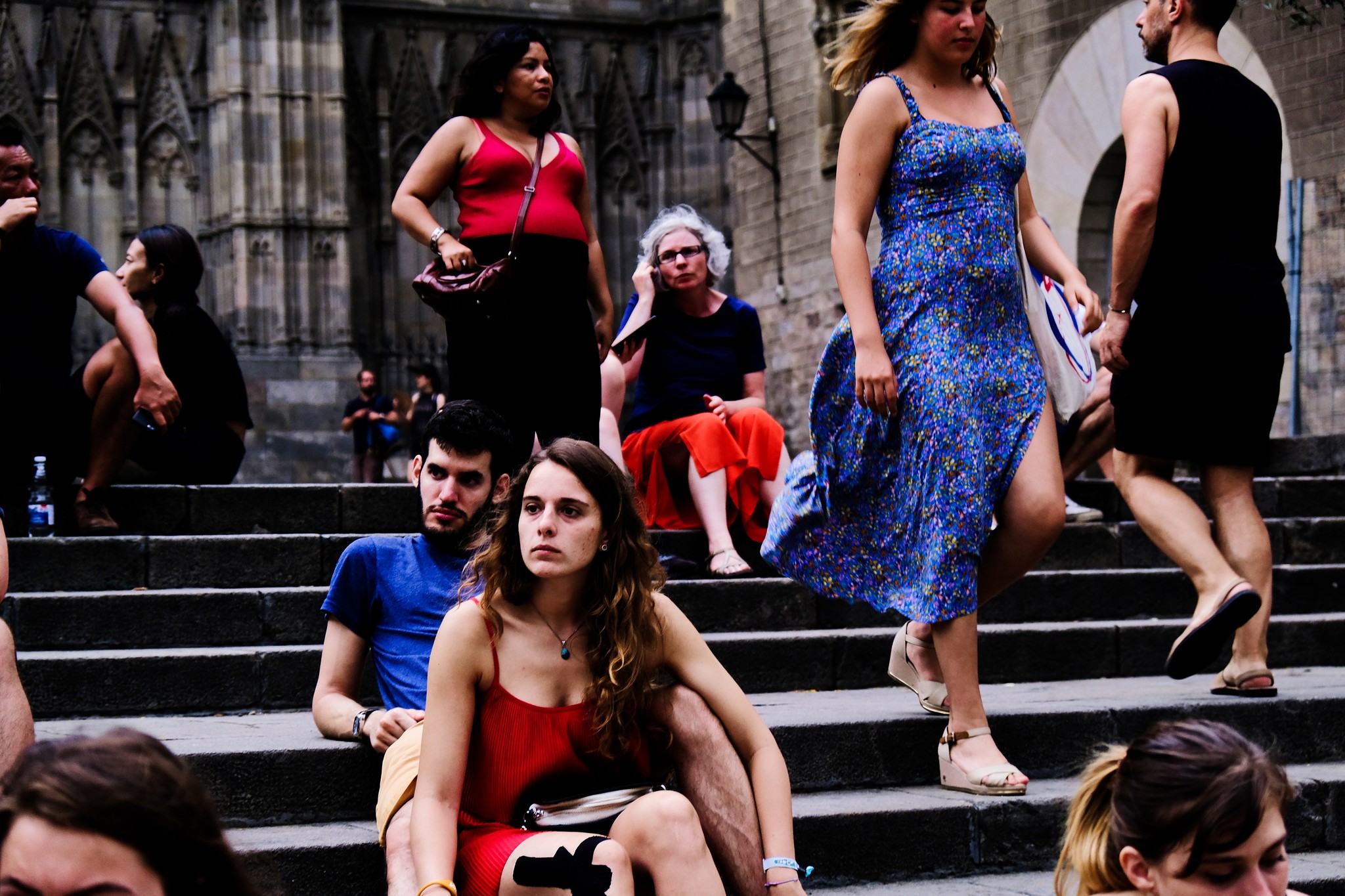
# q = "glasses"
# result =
<box><xmin>655</xmin><ymin>245</ymin><xmax>704</xmax><ymax>265</ymax></box>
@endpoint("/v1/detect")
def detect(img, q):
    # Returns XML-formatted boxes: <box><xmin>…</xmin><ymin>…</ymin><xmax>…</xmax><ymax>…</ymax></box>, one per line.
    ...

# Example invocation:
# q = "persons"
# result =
<box><xmin>0</xmin><ymin>0</ymin><xmax>1345</xmax><ymax>896</ymax></box>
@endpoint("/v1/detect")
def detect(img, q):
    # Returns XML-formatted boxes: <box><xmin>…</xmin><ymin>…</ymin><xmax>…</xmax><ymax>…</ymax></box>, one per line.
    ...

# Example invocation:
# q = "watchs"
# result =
<box><xmin>352</xmin><ymin>708</ymin><xmax>382</xmax><ymax>743</ymax></box>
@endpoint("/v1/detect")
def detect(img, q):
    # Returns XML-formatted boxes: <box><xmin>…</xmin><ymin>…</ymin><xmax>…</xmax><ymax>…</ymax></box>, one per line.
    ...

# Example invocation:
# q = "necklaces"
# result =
<box><xmin>531</xmin><ymin>599</ymin><xmax>590</xmax><ymax>660</ymax></box>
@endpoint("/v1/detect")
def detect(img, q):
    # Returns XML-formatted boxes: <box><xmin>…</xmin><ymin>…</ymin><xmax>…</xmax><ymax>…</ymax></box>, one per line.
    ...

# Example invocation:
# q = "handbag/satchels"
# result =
<box><xmin>1015</xmin><ymin>230</ymin><xmax>1097</xmax><ymax>424</ymax></box>
<box><xmin>519</xmin><ymin>786</ymin><xmax>668</xmax><ymax>832</ymax></box>
<box><xmin>412</xmin><ymin>240</ymin><xmax>508</xmax><ymax>320</ymax></box>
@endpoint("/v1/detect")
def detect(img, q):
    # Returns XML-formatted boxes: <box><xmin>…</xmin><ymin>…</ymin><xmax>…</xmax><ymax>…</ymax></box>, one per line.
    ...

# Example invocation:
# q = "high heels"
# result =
<box><xmin>887</xmin><ymin>621</ymin><xmax>952</xmax><ymax>715</ymax></box>
<box><xmin>938</xmin><ymin>725</ymin><xmax>1029</xmax><ymax>796</ymax></box>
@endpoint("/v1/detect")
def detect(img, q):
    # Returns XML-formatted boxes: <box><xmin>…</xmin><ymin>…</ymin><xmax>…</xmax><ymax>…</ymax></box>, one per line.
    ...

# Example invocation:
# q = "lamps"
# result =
<box><xmin>706</xmin><ymin>71</ymin><xmax>774</xmax><ymax>172</ymax></box>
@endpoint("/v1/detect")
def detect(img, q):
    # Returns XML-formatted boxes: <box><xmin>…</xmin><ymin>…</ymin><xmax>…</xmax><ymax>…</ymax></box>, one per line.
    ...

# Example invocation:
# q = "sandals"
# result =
<box><xmin>1210</xmin><ymin>668</ymin><xmax>1279</xmax><ymax>696</ymax></box>
<box><xmin>1166</xmin><ymin>577</ymin><xmax>1262</xmax><ymax>679</ymax></box>
<box><xmin>73</xmin><ymin>488</ymin><xmax>119</xmax><ymax>535</ymax></box>
<box><xmin>709</xmin><ymin>546</ymin><xmax>755</xmax><ymax>579</ymax></box>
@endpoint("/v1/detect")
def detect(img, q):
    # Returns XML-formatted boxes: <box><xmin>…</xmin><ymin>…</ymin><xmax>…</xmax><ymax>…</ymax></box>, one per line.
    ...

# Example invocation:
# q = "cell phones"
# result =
<box><xmin>132</xmin><ymin>407</ymin><xmax>158</xmax><ymax>432</ymax></box>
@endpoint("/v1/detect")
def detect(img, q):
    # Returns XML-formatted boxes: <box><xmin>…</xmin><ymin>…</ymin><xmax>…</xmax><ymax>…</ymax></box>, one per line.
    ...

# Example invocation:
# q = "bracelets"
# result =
<box><xmin>764</xmin><ymin>877</ymin><xmax>800</xmax><ymax>888</ymax></box>
<box><xmin>763</xmin><ymin>856</ymin><xmax>814</xmax><ymax>878</ymax></box>
<box><xmin>430</xmin><ymin>226</ymin><xmax>451</xmax><ymax>256</ymax></box>
<box><xmin>1108</xmin><ymin>304</ymin><xmax>1131</xmax><ymax>314</ymax></box>
<box><xmin>417</xmin><ymin>878</ymin><xmax>458</xmax><ymax>896</ymax></box>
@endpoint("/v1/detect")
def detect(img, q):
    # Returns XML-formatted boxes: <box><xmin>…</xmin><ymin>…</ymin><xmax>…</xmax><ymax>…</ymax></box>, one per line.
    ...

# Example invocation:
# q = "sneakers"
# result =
<box><xmin>1065</xmin><ymin>491</ymin><xmax>1103</xmax><ymax>523</ymax></box>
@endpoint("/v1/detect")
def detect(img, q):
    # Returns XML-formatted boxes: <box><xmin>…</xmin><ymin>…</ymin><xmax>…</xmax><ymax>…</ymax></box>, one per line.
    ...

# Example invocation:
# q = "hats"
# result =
<box><xmin>408</xmin><ymin>362</ymin><xmax>439</xmax><ymax>383</ymax></box>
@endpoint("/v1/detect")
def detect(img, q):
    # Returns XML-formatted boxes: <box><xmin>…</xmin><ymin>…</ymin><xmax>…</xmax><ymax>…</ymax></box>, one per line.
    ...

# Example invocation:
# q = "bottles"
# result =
<box><xmin>27</xmin><ymin>456</ymin><xmax>57</xmax><ymax>539</ymax></box>
